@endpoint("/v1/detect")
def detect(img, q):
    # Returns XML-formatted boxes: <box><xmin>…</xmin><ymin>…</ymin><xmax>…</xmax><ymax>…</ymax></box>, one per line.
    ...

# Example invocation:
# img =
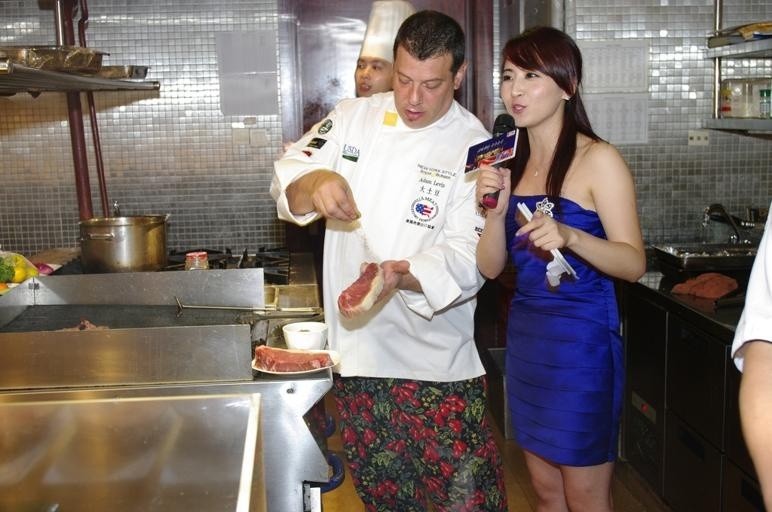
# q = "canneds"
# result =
<box><xmin>186</xmin><ymin>253</ymin><xmax>208</xmax><ymax>270</ymax></box>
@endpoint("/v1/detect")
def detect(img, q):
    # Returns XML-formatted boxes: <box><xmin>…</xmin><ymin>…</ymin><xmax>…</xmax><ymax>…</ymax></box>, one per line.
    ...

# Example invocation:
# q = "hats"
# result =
<box><xmin>358</xmin><ymin>0</ymin><xmax>419</xmax><ymax>66</ymax></box>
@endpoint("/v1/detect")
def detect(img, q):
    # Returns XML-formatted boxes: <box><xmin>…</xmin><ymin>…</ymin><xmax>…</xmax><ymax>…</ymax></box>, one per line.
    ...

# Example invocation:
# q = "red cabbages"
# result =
<box><xmin>32</xmin><ymin>262</ymin><xmax>54</xmax><ymax>274</ymax></box>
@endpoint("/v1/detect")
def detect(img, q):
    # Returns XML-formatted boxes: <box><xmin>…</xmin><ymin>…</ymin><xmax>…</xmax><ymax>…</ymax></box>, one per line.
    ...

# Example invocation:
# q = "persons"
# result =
<box><xmin>475</xmin><ymin>26</ymin><xmax>647</xmax><ymax>512</ymax></box>
<box><xmin>731</xmin><ymin>202</ymin><xmax>772</xmax><ymax>512</ymax></box>
<box><xmin>354</xmin><ymin>56</ymin><xmax>393</xmax><ymax>97</ymax></box>
<box><xmin>268</xmin><ymin>9</ymin><xmax>509</xmax><ymax>512</ymax></box>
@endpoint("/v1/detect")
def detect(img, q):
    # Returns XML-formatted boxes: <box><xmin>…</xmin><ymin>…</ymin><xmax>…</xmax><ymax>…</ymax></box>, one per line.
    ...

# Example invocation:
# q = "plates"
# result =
<box><xmin>252</xmin><ymin>349</ymin><xmax>341</xmax><ymax>376</ymax></box>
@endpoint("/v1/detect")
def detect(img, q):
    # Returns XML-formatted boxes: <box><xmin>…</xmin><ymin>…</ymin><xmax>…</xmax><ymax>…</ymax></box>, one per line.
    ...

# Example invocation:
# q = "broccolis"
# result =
<box><xmin>0</xmin><ymin>257</ymin><xmax>15</xmax><ymax>282</ymax></box>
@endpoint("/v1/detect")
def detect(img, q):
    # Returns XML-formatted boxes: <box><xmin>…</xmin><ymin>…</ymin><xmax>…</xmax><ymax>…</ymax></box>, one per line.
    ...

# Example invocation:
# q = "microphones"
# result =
<box><xmin>482</xmin><ymin>109</ymin><xmax>515</xmax><ymax>210</ymax></box>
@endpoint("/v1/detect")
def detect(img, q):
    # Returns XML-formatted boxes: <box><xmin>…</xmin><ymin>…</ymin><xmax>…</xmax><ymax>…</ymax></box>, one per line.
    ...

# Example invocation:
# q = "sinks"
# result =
<box><xmin>650</xmin><ymin>239</ymin><xmax>759</xmax><ymax>274</ymax></box>
<box><xmin>0</xmin><ymin>392</ymin><xmax>266</xmax><ymax>512</ymax></box>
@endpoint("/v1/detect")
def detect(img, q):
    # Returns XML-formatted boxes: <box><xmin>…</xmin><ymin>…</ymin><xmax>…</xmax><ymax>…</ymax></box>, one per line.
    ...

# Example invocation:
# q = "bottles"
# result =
<box><xmin>183</xmin><ymin>251</ymin><xmax>210</xmax><ymax>272</ymax></box>
<box><xmin>720</xmin><ymin>77</ymin><xmax>772</xmax><ymax>120</ymax></box>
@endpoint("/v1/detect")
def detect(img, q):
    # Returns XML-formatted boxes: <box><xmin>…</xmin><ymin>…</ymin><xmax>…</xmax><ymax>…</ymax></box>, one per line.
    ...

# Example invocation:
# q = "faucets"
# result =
<box><xmin>702</xmin><ymin>204</ymin><xmax>741</xmax><ymax>240</ymax></box>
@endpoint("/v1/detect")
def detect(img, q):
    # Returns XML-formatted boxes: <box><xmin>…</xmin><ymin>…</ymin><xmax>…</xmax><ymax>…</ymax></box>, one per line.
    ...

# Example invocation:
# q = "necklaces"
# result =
<box><xmin>534</xmin><ymin>170</ymin><xmax>538</xmax><ymax>177</ymax></box>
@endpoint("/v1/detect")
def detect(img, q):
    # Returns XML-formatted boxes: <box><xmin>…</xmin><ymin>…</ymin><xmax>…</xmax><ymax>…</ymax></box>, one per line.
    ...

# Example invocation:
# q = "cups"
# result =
<box><xmin>281</xmin><ymin>321</ymin><xmax>328</xmax><ymax>353</ymax></box>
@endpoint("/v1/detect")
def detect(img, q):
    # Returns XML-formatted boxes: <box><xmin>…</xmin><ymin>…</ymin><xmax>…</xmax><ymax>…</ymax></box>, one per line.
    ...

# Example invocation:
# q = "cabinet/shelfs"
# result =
<box><xmin>698</xmin><ymin>0</ymin><xmax>772</xmax><ymax>132</ymax></box>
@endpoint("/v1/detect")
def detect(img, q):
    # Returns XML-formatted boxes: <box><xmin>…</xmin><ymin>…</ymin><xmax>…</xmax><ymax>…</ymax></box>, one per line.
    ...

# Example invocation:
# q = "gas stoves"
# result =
<box><xmin>55</xmin><ymin>248</ymin><xmax>293</xmax><ymax>283</ymax></box>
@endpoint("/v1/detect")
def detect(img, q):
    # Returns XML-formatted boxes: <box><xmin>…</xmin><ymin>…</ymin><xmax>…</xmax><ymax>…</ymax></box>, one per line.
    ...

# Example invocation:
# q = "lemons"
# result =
<box><xmin>12</xmin><ymin>257</ymin><xmax>39</xmax><ymax>282</ymax></box>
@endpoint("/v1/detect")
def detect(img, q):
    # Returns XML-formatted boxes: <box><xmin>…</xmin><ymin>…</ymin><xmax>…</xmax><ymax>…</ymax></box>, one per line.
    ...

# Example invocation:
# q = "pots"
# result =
<box><xmin>73</xmin><ymin>213</ymin><xmax>170</xmax><ymax>274</ymax></box>
